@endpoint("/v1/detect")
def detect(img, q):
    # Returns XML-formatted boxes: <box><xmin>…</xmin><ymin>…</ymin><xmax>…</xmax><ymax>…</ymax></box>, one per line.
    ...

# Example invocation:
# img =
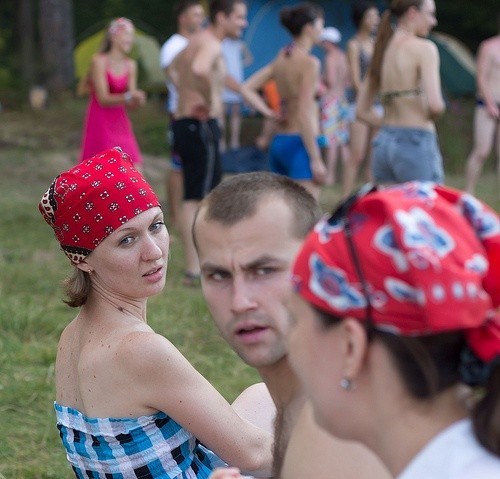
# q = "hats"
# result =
<box><xmin>38</xmin><ymin>146</ymin><xmax>160</xmax><ymax>265</ymax></box>
<box><xmin>320</xmin><ymin>27</ymin><xmax>342</xmax><ymax>43</ymax></box>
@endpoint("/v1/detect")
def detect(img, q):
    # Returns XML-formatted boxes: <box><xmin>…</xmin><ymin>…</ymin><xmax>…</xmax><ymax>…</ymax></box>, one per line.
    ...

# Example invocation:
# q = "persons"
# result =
<box><xmin>191</xmin><ymin>173</ymin><xmax>391</xmax><ymax>479</ymax></box>
<box><xmin>77</xmin><ymin>16</ymin><xmax>147</xmax><ymax>184</ymax></box>
<box><xmin>284</xmin><ymin>179</ymin><xmax>500</xmax><ymax>479</ymax></box>
<box><xmin>160</xmin><ymin>0</ymin><xmax>447</xmax><ymax>284</ymax></box>
<box><xmin>465</xmin><ymin>33</ymin><xmax>500</xmax><ymax>195</ymax></box>
<box><xmin>38</xmin><ymin>146</ymin><xmax>277</xmax><ymax>479</ymax></box>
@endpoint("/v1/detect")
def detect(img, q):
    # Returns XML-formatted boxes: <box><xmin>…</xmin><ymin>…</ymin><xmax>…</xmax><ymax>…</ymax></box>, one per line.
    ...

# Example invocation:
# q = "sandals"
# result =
<box><xmin>184</xmin><ymin>273</ymin><xmax>200</xmax><ymax>288</ymax></box>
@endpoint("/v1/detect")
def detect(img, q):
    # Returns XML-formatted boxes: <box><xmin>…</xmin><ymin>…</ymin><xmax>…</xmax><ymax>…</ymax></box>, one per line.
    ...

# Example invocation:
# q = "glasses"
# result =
<box><xmin>327</xmin><ymin>182</ymin><xmax>414</xmax><ymax>344</ymax></box>
